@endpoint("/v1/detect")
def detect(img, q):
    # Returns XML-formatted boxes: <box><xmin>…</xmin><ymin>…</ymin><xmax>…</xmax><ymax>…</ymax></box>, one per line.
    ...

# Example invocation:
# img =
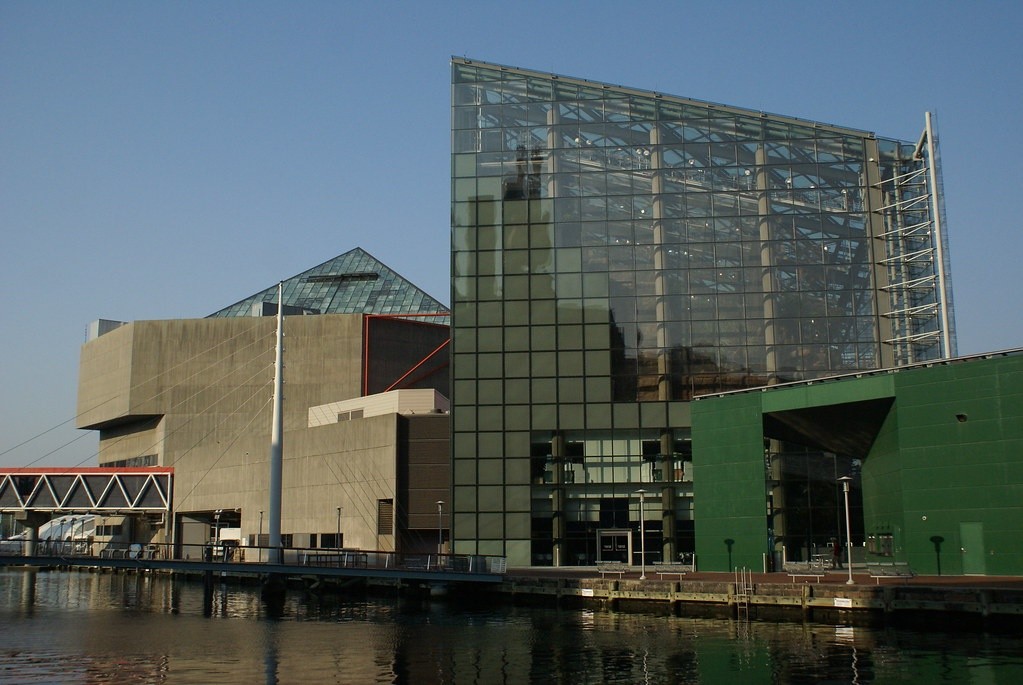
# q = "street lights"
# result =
<box><xmin>838</xmin><ymin>476</ymin><xmax>857</xmax><ymax>584</ymax></box>
<box><xmin>258</xmin><ymin>510</ymin><xmax>265</xmax><ymax>561</ymax></box>
<box><xmin>635</xmin><ymin>489</ymin><xmax>649</xmax><ymax>579</ymax></box>
<box><xmin>336</xmin><ymin>506</ymin><xmax>344</xmax><ymax>567</ymax></box>
<box><xmin>436</xmin><ymin>501</ymin><xmax>446</xmax><ymax>567</ymax></box>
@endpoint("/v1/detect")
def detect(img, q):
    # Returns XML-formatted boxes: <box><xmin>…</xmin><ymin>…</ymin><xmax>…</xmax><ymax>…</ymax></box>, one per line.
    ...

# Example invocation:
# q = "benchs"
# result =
<box><xmin>785</xmin><ymin>562</ymin><xmax>825</xmax><ymax>583</ymax></box>
<box><xmin>867</xmin><ymin>563</ymin><xmax>913</xmax><ymax>585</ymax></box>
<box><xmin>596</xmin><ymin>561</ymin><xmax>625</xmax><ymax>579</ymax></box>
<box><xmin>653</xmin><ymin>561</ymin><xmax>686</xmax><ymax>580</ymax></box>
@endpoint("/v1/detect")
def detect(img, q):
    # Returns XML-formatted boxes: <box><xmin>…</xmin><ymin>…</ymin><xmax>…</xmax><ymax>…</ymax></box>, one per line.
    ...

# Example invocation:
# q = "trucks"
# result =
<box><xmin>212</xmin><ymin>527</ymin><xmax>242</xmax><ymax>560</ymax></box>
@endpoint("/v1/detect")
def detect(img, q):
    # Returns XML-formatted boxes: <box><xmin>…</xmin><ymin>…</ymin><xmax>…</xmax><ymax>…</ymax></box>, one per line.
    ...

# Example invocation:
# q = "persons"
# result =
<box><xmin>832</xmin><ymin>535</ymin><xmax>844</xmax><ymax>570</ymax></box>
